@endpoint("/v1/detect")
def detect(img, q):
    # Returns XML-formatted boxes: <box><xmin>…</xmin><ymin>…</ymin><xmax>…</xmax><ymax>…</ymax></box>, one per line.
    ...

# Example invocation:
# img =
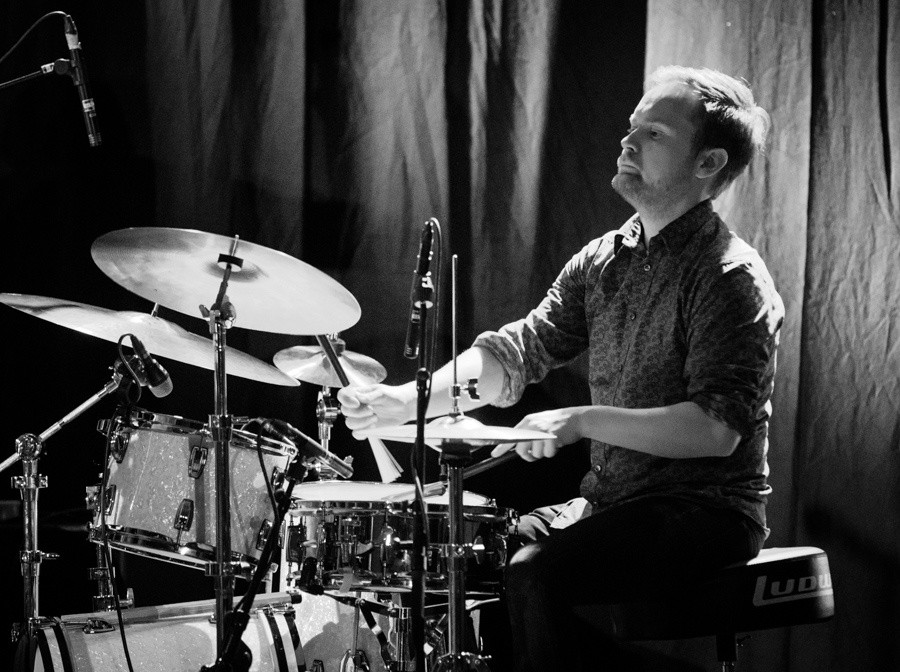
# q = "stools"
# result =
<box><xmin>622</xmin><ymin>546</ymin><xmax>835</xmax><ymax>672</ymax></box>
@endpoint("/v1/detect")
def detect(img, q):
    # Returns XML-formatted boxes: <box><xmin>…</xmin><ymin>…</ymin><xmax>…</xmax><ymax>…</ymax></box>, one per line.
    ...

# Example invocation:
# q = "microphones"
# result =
<box><xmin>255</xmin><ymin>418</ymin><xmax>354</xmax><ymax>479</ymax></box>
<box><xmin>402</xmin><ymin>221</ymin><xmax>434</xmax><ymax>360</ymax></box>
<box><xmin>63</xmin><ymin>14</ymin><xmax>102</xmax><ymax>147</ymax></box>
<box><xmin>130</xmin><ymin>334</ymin><xmax>172</xmax><ymax>399</ymax></box>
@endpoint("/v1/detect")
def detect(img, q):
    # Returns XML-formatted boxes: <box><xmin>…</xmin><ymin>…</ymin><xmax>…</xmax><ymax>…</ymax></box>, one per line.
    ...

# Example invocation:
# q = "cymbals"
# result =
<box><xmin>273</xmin><ymin>345</ymin><xmax>388</xmax><ymax>389</ymax></box>
<box><xmin>91</xmin><ymin>228</ymin><xmax>362</xmax><ymax>336</ymax></box>
<box><xmin>0</xmin><ymin>293</ymin><xmax>301</xmax><ymax>388</ymax></box>
<box><xmin>362</xmin><ymin>416</ymin><xmax>558</xmax><ymax>439</ymax></box>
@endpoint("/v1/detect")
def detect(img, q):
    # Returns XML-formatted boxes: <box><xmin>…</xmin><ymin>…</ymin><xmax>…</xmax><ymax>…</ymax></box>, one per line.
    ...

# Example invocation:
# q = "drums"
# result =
<box><xmin>85</xmin><ymin>407</ymin><xmax>296</xmax><ymax>581</ymax></box>
<box><xmin>288</xmin><ymin>480</ymin><xmax>517</xmax><ymax>595</ymax></box>
<box><xmin>26</xmin><ymin>591</ymin><xmax>309</xmax><ymax>672</ymax></box>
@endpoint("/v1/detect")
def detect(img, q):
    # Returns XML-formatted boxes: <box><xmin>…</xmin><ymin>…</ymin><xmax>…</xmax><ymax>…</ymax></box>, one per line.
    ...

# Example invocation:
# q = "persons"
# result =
<box><xmin>337</xmin><ymin>66</ymin><xmax>786</xmax><ymax>672</ymax></box>
